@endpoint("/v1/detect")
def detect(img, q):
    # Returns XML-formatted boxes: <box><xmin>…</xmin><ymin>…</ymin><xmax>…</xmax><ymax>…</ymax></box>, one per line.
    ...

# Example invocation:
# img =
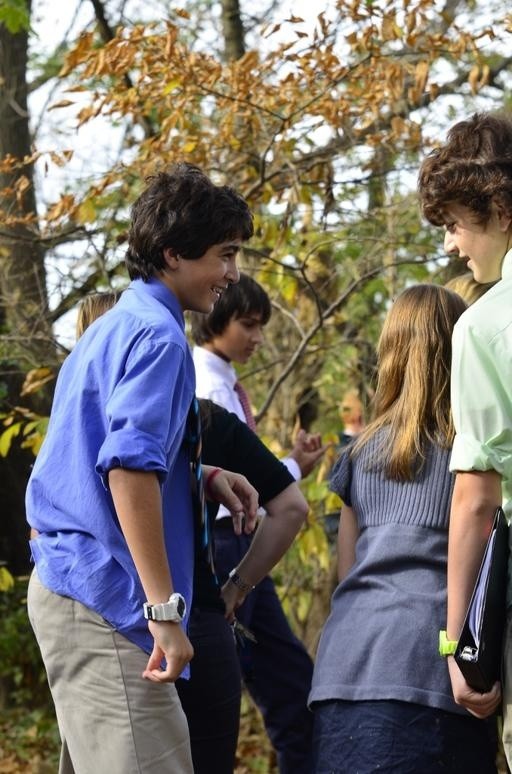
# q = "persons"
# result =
<box><xmin>417</xmin><ymin>110</ymin><xmax>512</xmax><ymax>773</ymax></box>
<box><xmin>189</xmin><ymin>272</ymin><xmax>334</xmax><ymax>774</ymax></box>
<box><xmin>22</xmin><ymin>158</ymin><xmax>252</xmax><ymax>774</ymax></box>
<box><xmin>322</xmin><ymin>384</ymin><xmax>379</xmax><ymax>555</ymax></box>
<box><xmin>303</xmin><ymin>284</ymin><xmax>502</xmax><ymax>773</ymax></box>
<box><xmin>440</xmin><ymin>273</ymin><xmax>494</xmax><ymax>309</ymax></box>
<box><xmin>74</xmin><ymin>291</ymin><xmax>311</xmax><ymax>774</ymax></box>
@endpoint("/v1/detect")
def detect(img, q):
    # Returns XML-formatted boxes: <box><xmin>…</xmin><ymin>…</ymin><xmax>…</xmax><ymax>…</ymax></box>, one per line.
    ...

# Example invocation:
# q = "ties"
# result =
<box><xmin>233</xmin><ymin>384</ymin><xmax>255</xmax><ymax>432</ymax></box>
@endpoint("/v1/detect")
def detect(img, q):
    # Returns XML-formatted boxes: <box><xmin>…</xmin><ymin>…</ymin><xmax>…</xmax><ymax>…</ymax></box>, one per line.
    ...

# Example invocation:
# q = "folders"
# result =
<box><xmin>454</xmin><ymin>506</ymin><xmax>509</xmax><ymax>716</ymax></box>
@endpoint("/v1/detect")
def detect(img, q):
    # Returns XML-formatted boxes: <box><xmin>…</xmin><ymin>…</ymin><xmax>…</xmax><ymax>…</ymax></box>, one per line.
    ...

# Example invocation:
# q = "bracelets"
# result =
<box><xmin>204</xmin><ymin>463</ymin><xmax>225</xmax><ymax>502</ymax></box>
<box><xmin>227</xmin><ymin>568</ymin><xmax>254</xmax><ymax>596</ymax></box>
<box><xmin>435</xmin><ymin>628</ymin><xmax>462</xmax><ymax>656</ymax></box>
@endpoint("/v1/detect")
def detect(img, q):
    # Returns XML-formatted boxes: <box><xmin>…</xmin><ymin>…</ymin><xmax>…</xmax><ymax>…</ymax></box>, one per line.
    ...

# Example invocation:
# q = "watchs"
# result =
<box><xmin>139</xmin><ymin>591</ymin><xmax>187</xmax><ymax>627</ymax></box>
